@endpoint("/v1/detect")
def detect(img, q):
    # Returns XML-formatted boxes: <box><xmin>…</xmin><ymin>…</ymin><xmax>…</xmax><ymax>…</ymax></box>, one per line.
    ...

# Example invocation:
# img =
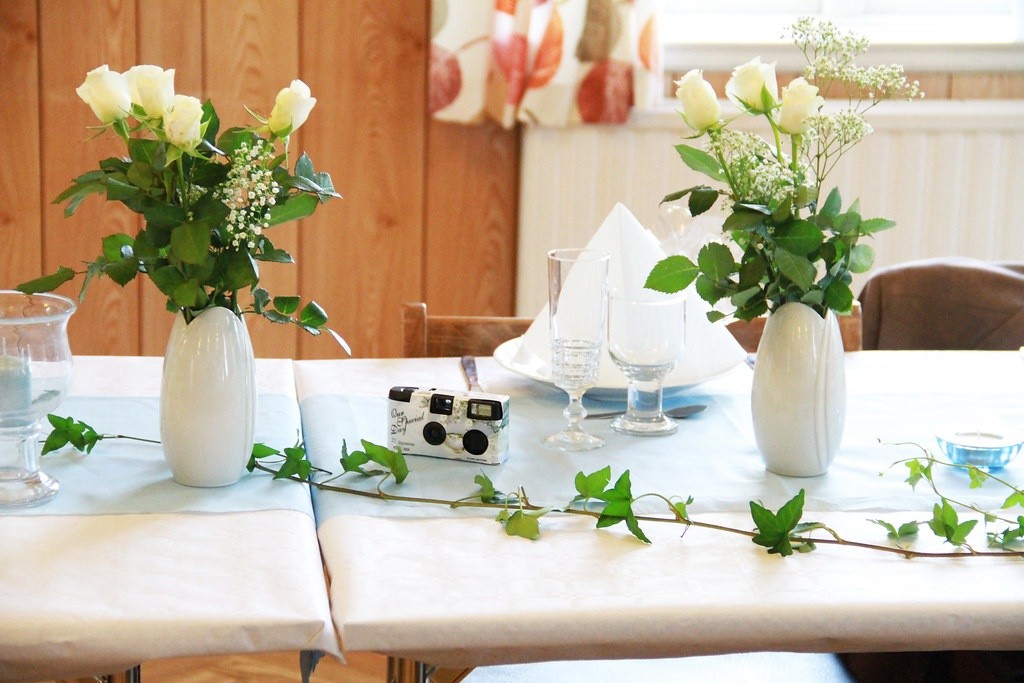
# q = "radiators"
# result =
<box><xmin>517</xmin><ymin>97</ymin><xmax>1024</xmax><ymax>324</ymax></box>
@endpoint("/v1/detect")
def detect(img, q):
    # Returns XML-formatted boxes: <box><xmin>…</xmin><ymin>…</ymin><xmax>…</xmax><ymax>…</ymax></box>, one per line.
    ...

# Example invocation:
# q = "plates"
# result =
<box><xmin>494</xmin><ymin>333</ymin><xmax>746</xmax><ymax>402</ymax></box>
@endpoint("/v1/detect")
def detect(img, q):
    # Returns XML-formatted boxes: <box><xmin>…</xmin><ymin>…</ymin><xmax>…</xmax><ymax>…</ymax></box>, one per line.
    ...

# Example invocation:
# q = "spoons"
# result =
<box><xmin>580</xmin><ymin>403</ymin><xmax>705</xmax><ymax>420</ymax></box>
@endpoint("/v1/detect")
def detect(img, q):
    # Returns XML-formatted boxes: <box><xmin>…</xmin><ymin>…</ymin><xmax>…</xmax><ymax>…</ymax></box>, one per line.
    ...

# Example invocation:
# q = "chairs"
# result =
<box><xmin>856</xmin><ymin>259</ymin><xmax>1024</xmax><ymax>353</ymax></box>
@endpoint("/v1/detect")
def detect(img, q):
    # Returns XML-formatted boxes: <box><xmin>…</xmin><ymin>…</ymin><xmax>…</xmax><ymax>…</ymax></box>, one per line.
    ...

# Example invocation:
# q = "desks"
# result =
<box><xmin>2</xmin><ymin>353</ymin><xmax>343</xmax><ymax>669</ymax></box>
<box><xmin>295</xmin><ymin>349</ymin><xmax>1024</xmax><ymax>652</ymax></box>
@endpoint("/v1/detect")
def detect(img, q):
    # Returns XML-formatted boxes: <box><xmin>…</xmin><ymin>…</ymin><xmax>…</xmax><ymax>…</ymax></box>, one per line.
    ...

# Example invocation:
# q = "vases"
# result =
<box><xmin>159</xmin><ymin>296</ymin><xmax>258</xmax><ymax>488</ymax></box>
<box><xmin>750</xmin><ymin>301</ymin><xmax>849</xmax><ymax>479</ymax></box>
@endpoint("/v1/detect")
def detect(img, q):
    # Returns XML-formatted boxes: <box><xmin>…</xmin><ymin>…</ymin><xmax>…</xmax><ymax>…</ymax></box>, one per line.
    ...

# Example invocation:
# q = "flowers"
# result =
<box><xmin>639</xmin><ymin>17</ymin><xmax>927</xmax><ymax>318</ymax></box>
<box><xmin>51</xmin><ymin>61</ymin><xmax>354</xmax><ymax>358</ymax></box>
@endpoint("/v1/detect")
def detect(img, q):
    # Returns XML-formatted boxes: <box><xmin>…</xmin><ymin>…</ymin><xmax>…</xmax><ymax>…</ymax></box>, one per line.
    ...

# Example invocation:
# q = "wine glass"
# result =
<box><xmin>606</xmin><ymin>260</ymin><xmax>688</xmax><ymax>435</ymax></box>
<box><xmin>2</xmin><ymin>288</ymin><xmax>75</xmax><ymax>507</ymax></box>
<box><xmin>548</xmin><ymin>249</ymin><xmax>603</xmax><ymax>453</ymax></box>
